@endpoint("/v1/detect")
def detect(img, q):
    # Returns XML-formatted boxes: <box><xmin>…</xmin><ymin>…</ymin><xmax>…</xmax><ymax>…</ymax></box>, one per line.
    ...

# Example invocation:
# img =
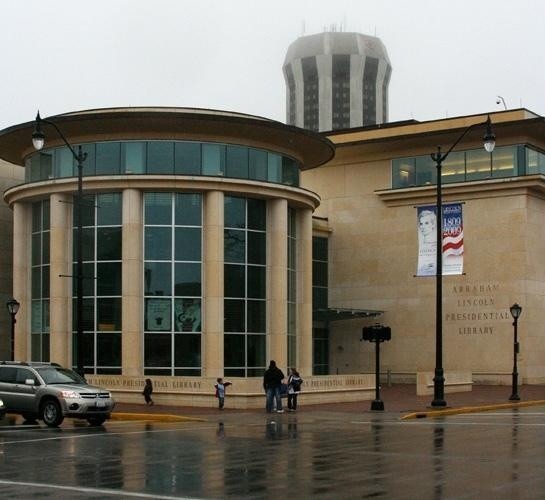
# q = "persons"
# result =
<box><xmin>286</xmin><ymin>371</ymin><xmax>300</xmax><ymax>390</ymax></box>
<box><xmin>213</xmin><ymin>377</ymin><xmax>232</xmax><ymax>409</ymax></box>
<box><xmin>284</xmin><ymin>366</ymin><xmax>302</xmax><ymax>412</ymax></box>
<box><xmin>263</xmin><ymin>359</ymin><xmax>284</xmax><ymax>414</ymax></box>
<box><xmin>418</xmin><ymin>210</ymin><xmax>437</xmax><ymax>239</ymax></box>
<box><xmin>140</xmin><ymin>378</ymin><xmax>154</xmax><ymax>406</ymax></box>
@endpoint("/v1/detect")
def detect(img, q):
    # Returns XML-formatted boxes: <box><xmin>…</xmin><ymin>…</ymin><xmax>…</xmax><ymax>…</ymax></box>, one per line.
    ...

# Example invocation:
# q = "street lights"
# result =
<box><xmin>509</xmin><ymin>302</ymin><xmax>522</xmax><ymax>400</ymax></box>
<box><xmin>7</xmin><ymin>299</ymin><xmax>20</xmax><ymax>361</ymax></box>
<box><xmin>430</xmin><ymin>112</ymin><xmax>497</xmax><ymax>408</ymax></box>
<box><xmin>496</xmin><ymin>95</ymin><xmax>508</xmax><ymax>110</ymax></box>
<box><xmin>31</xmin><ymin>109</ymin><xmax>88</xmax><ymax>378</ymax></box>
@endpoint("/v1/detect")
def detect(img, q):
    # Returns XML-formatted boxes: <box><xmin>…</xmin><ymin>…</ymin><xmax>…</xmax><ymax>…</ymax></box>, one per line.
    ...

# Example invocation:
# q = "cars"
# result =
<box><xmin>1</xmin><ymin>360</ymin><xmax>115</xmax><ymax>428</ymax></box>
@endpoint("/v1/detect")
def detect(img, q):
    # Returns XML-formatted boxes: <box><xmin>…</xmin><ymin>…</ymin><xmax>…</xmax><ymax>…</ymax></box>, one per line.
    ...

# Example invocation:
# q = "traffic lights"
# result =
<box><xmin>360</xmin><ymin>323</ymin><xmax>391</xmax><ymax>344</ymax></box>
